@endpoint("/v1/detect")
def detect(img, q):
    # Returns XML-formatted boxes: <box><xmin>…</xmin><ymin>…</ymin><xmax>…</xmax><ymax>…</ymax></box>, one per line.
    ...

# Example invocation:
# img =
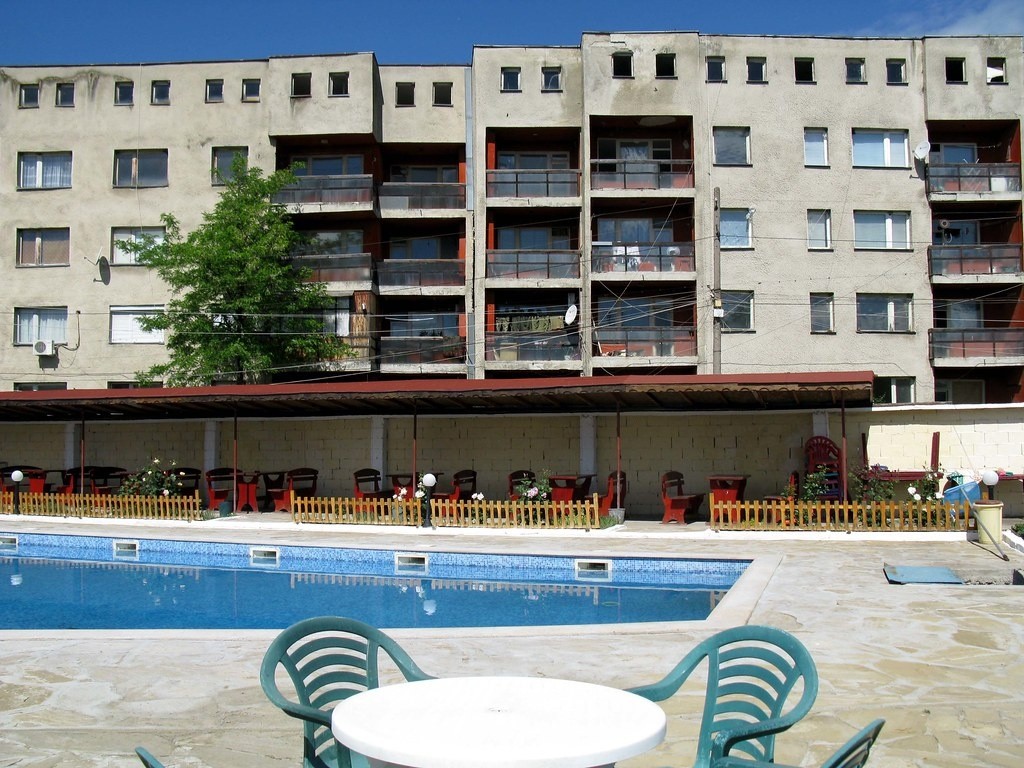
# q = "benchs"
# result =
<box><xmin>0</xmin><ymin>465</ymin><xmax>48</xmax><ymax>504</ymax></box>
<box><xmin>762</xmin><ymin>471</ymin><xmax>800</xmax><ymax>524</ymax></box>
<box><xmin>267</xmin><ymin>467</ymin><xmax>320</xmax><ymax>513</ymax></box>
<box><xmin>660</xmin><ymin>471</ymin><xmax>707</xmax><ymax>525</ymax></box>
<box><xmin>352</xmin><ymin>468</ymin><xmax>394</xmax><ymax>515</ymax></box>
<box><xmin>432</xmin><ymin>470</ymin><xmax>477</xmax><ymax>517</ymax></box>
<box><xmin>89</xmin><ymin>466</ymin><xmax>127</xmax><ymax>507</ymax></box>
<box><xmin>507</xmin><ymin>470</ymin><xmax>543</xmax><ymax>521</ymax></box>
<box><xmin>50</xmin><ymin>466</ymin><xmax>106</xmax><ymax>506</ymax></box>
<box><xmin>159</xmin><ymin>467</ymin><xmax>203</xmax><ymax>508</ymax></box>
<box><xmin>584</xmin><ymin>470</ymin><xmax>627</xmax><ymax>521</ymax></box>
<box><xmin>204</xmin><ymin>467</ymin><xmax>244</xmax><ymax>511</ymax></box>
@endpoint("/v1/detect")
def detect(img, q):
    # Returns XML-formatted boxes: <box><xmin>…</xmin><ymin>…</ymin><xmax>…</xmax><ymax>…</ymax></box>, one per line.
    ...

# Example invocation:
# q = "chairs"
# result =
<box><xmin>600</xmin><ymin>623</ymin><xmax>820</xmax><ymax>768</ymax></box>
<box><xmin>260</xmin><ymin>614</ymin><xmax>439</xmax><ymax>768</ymax></box>
<box><xmin>802</xmin><ymin>435</ymin><xmax>843</xmax><ymax>522</ymax></box>
<box><xmin>712</xmin><ymin>717</ymin><xmax>887</xmax><ymax>768</ymax></box>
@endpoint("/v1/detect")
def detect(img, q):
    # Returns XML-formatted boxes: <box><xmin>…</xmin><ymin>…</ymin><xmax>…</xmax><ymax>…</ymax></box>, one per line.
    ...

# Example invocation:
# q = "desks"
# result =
<box><xmin>999</xmin><ymin>474</ymin><xmax>1024</xmax><ymax>481</ymax></box>
<box><xmin>704</xmin><ymin>474</ymin><xmax>752</xmax><ymax>524</ymax></box>
<box><xmin>858</xmin><ymin>431</ymin><xmax>945</xmax><ymax>503</ymax></box>
<box><xmin>384</xmin><ymin>472</ymin><xmax>445</xmax><ymax>515</ymax></box>
<box><xmin>21</xmin><ymin>468</ymin><xmax>67</xmax><ymax>501</ymax></box>
<box><xmin>230</xmin><ymin>470</ymin><xmax>289</xmax><ymax>513</ymax></box>
<box><xmin>119</xmin><ymin>471</ymin><xmax>138</xmax><ymax>475</ymax></box>
<box><xmin>546</xmin><ymin>473</ymin><xmax>598</xmax><ymax>519</ymax></box>
<box><xmin>332</xmin><ymin>675</ymin><xmax>667</xmax><ymax>768</ymax></box>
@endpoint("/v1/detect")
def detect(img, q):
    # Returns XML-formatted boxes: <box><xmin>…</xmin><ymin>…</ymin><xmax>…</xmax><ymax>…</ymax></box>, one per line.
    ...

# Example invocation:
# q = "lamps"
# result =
<box><xmin>983</xmin><ymin>470</ymin><xmax>998</xmax><ymax>500</ymax></box>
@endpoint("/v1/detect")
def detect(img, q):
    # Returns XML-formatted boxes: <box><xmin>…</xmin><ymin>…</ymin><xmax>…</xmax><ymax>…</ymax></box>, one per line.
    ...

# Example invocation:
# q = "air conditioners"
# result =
<box><xmin>33</xmin><ymin>340</ymin><xmax>56</xmax><ymax>356</ymax></box>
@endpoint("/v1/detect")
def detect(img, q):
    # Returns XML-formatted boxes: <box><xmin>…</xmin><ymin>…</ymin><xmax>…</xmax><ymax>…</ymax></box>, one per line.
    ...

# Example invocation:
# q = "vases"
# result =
<box><xmin>606</xmin><ymin>508</ymin><xmax>626</xmax><ymax>525</ymax></box>
